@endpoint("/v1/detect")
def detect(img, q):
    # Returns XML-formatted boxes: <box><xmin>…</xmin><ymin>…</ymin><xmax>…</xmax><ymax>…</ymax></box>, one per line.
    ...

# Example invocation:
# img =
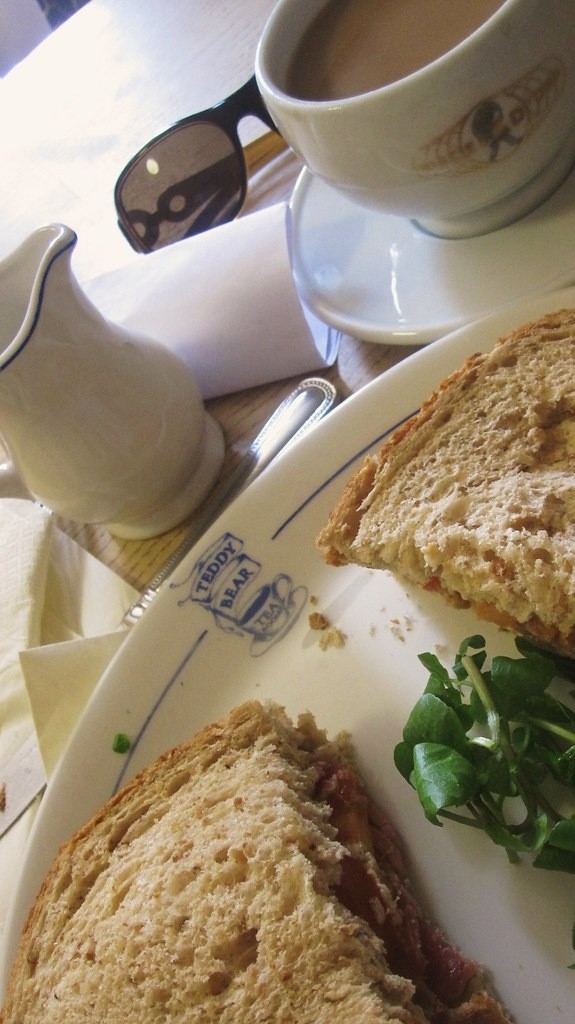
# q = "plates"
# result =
<box><xmin>288</xmin><ymin>164</ymin><xmax>575</xmax><ymax>347</ymax></box>
<box><xmin>0</xmin><ymin>289</ymin><xmax>574</xmax><ymax>1024</ymax></box>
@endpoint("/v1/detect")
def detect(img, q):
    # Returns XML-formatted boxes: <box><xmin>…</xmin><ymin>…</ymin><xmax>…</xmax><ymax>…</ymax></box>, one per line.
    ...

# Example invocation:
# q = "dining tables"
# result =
<box><xmin>0</xmin><ymin>0</ymin><xmax>412</xmax><ymax>587</ymax></box>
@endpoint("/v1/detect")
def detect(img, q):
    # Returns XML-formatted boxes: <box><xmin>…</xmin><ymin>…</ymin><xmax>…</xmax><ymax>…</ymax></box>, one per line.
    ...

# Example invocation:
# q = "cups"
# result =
<box><xmin>255</xmin><ymin>0</ymin><xmax>575</xmax><ymax>241</ymax></box>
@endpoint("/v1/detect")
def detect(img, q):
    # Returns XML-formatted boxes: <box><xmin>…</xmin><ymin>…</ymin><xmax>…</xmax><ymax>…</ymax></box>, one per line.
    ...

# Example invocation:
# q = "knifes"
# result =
<box><xmin>0</xmin><ymin>376</ymin><xmax>337</xmax><ymax>837</ymax></box>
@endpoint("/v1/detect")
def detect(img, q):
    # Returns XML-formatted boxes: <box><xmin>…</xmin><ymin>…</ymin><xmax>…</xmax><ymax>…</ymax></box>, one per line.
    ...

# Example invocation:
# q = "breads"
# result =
<box><xmin>2</xmin><ymin>696</ymin><xmax>514</xmax><ymax>1024</ymax></box>
<box><xmin>318</xmin><ymin>308</ymin><xmax>575</xmax><ymax>648</ymax></box>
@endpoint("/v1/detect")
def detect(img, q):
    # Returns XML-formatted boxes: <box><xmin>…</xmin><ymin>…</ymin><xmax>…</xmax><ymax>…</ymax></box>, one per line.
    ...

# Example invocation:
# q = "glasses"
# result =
<box><xmin>110</xmin><ymin>74</ymin><xmax>289</xmax><ymax>256</ymax></box>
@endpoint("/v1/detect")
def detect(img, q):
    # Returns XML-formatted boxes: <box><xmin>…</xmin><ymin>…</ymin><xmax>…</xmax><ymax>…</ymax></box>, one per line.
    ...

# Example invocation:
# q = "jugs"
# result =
<box><xmin>1</xmin><ymin>224</ymin><xmax>226</xmax><ymax>541</ymax></box>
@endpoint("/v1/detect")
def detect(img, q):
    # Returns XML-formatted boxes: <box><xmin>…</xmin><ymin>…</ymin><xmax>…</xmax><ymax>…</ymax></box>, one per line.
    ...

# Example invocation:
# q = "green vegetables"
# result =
<box><xmin>393</xmin><ymin>634</ymin><xmax>575</xmax><ymax>874</ymax></box>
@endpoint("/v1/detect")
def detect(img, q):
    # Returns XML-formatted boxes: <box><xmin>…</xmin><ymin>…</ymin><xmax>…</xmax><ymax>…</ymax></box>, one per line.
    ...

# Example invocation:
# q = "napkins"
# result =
<box><xmin>0</xmin><ymin>497</ymin><xmax>141</xmax><ymax>837</ymax></box>
